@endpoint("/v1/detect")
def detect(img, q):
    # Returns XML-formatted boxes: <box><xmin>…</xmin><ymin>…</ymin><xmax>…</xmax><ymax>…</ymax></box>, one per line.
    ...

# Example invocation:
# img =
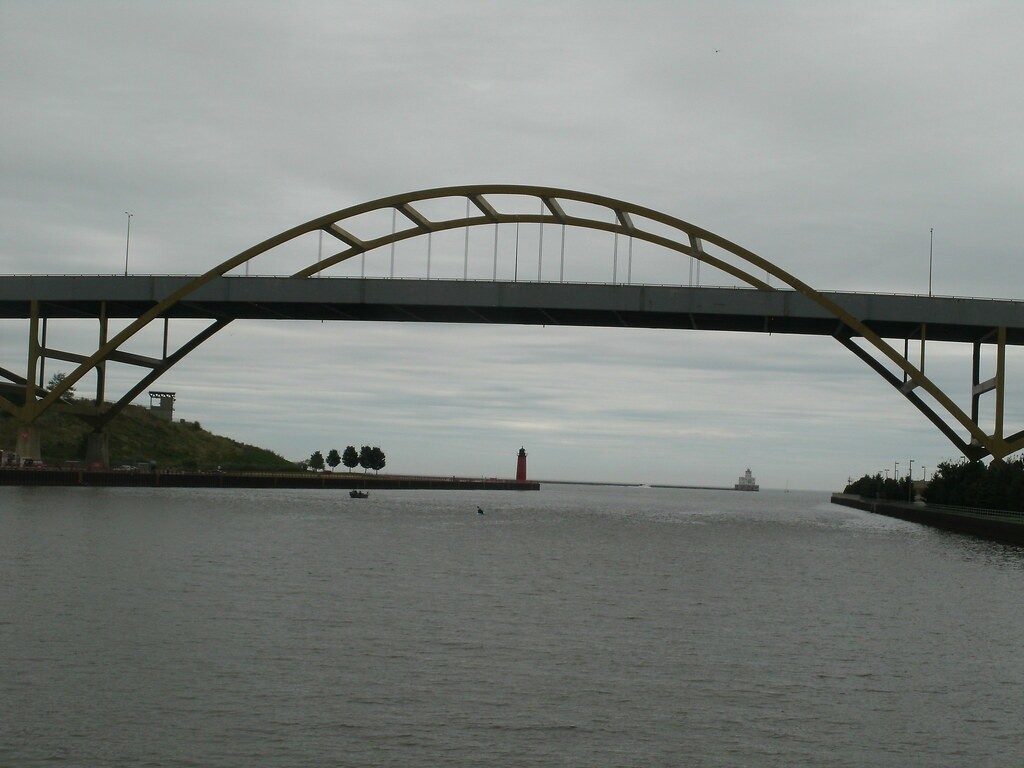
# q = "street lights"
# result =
<box><xmin>909</xmin><ymin>469</ymin><xmax>912</xmax><ymax>481</ymax></box>
<box><xmin>885</xmin><ymin>469</ymin><xmax>889</xmax><ymax>480</ymax></box>
<box><xmin>125</xmin><ymin>211</ymin><xmax>134</xmax><ymax>276</ymax></box>
<box><xmin>960</xmin><ymin>456</ymin><xmax>965</xmax><ymax>465</ymax></box>
<box><xmin>922</xmin><ymin>466</ymin><xmax>927</xmax><ymax>480</ymax></box>
<box><xmin>909</xmin><ymin>460</ymin><xmax>915</xmax><ymax>503</ymax></box>
<box><xmin>894</xmin><ymin>461</ymin><xmax>900</xmax><ymax>479</ymax></box>
<box><xmin>896</xmin><ymin>470</ymin><xmax>899</xmax><ymax>482</ymax></box>
<box><xmin>929</xmin><ymin>227</ymin><xmax>933</xmax><ymax>297</ymax></box>
<box><xmin>880</xmin><ymin>470</ymin><xmax>883</xmax><ymax>480</ymax></box>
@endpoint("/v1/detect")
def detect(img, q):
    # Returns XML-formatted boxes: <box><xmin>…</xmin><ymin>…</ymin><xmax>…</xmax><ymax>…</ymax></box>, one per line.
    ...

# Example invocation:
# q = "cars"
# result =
<box><xmin>112</xmin><ymin>465</ymin><xmax>138</xmax><ymax>471</ymax></box>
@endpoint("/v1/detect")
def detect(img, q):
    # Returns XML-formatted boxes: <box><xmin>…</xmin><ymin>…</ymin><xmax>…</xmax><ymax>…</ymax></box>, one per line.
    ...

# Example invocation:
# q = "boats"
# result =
<box><xmin>735</xmin><ymin>468</ymin><xmax>759</xmax><ymax>491</ymax></box>
<box><xmin>783</xmin><ymin>489</ymin><xmax>791</xmax><ymax>495</ymax></box>
<box><xmin>349</xmin><ymin>491</ymin><xmax>369</xmax><ymax>498</ymax></box>
